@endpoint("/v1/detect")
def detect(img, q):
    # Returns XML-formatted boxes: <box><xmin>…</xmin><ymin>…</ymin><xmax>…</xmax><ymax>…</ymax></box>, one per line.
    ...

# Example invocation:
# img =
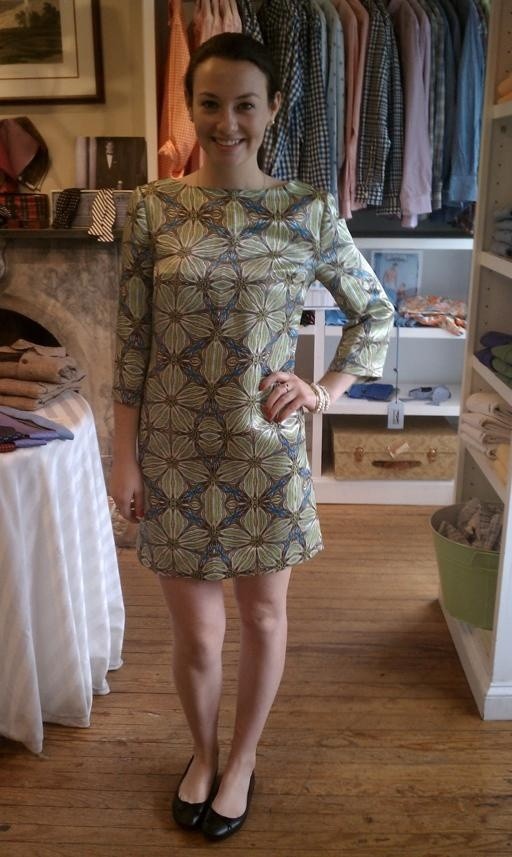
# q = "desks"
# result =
<box><xmin>1</xmin><ymin>389</ymin><xmax>126</xmax><ymax>754</ymax></box>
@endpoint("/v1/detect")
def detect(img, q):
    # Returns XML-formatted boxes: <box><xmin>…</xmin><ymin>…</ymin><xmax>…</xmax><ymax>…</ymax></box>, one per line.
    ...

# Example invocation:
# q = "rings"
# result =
<box><xmin>284</xmin><ymin>382</ymin><xmax>289</xmax><ymax>392</ymax></box>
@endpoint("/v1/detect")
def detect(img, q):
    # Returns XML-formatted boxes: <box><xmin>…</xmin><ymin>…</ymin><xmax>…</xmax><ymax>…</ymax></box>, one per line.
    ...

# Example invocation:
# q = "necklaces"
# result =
<box><xmin>196</xmin><ymin>166</ymin><xmax>266</xmax><ymax>191</ymax></box>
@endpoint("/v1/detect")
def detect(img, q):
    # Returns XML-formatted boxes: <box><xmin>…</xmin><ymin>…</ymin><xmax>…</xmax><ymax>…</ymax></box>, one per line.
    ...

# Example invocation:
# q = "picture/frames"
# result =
<box><xmin>0</xmin><ymin>0</ymin><xmax>107</xmax><ymax>106</ymax></box>
<box><xmin>371</xmin><ymin>250</ymin><xmax>423</xmax><ymax>305</ymax></box>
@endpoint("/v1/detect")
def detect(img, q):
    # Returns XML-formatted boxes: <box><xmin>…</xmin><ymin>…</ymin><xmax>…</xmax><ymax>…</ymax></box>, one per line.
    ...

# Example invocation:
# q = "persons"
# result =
<box><xmin>98</xmin><ymin>141</ymin><xmax>124</xmax><ymax>190</ymax></box>
<box><xmin>107</xmin><ymin>33</ymin><xmax>397</xmax><ymax>842</ymax></box>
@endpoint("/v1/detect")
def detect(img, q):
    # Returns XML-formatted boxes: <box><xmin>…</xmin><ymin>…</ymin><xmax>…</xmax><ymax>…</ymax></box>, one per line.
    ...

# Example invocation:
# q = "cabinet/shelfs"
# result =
<box><xmin>143</xmin><ymin>1</ymin><xmax>474</xmax><ymax>507</ymax></box>
<box><xmin>437</xmin><ymin>1</ymin><xmax>512</xmax><ymax>720</ymax></box>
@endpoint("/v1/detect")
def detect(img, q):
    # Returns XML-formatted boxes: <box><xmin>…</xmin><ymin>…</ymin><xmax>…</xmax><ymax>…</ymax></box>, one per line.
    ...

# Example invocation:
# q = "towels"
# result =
<box><xmin>459</xmin><ymin>391</ymin><xmax>511</xmax><ymax>488</ymax></box>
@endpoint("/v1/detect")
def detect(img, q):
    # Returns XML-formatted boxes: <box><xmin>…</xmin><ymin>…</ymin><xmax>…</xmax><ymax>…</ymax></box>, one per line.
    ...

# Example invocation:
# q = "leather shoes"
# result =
<box><xmin>172</xmin><ymin>755</ymin><xmax>215</xmax><ymax>828</ymax></box>
<box><xmin>202</xmin><ymin>770</ymin><xmax>255</xmax><ymax>841</ymax></box>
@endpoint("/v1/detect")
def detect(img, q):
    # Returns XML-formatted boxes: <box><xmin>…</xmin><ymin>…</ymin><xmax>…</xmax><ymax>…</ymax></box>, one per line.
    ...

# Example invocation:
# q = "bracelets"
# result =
<box><xmin>310</xmin><ymin>382</ymin><xmax>330</xmax><ymax>414</ymax></box>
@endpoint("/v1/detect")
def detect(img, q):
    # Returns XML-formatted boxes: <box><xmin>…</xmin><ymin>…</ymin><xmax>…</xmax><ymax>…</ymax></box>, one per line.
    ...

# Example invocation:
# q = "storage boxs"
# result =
<box><xmin>328</xmin><ymin>416</ymin><xmax>459</xmax><ymax>482</ymax></box>
